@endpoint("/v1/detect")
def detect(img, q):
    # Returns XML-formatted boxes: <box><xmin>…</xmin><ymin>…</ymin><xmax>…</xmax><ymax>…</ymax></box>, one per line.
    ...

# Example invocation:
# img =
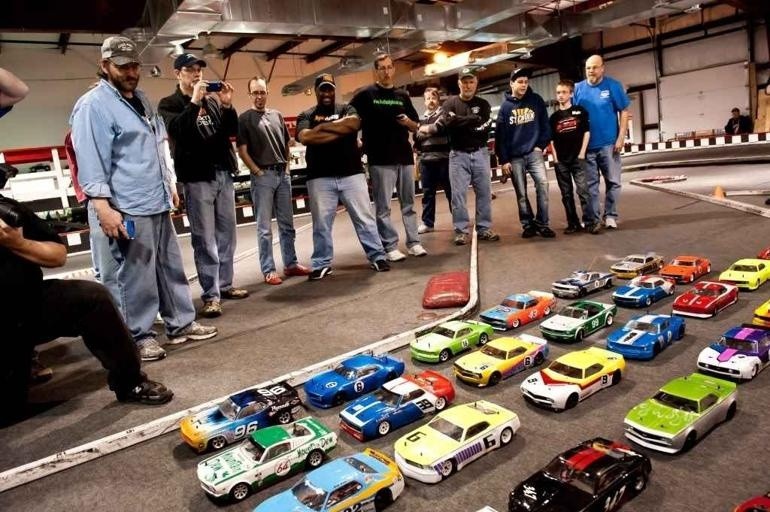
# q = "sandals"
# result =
<box><xmin>117</xmin><ymin>380</ymin><xmax>175</xmax><ymax>405</ymax></box>
<box><xmin>31</xmin><ymin>352</ymin><xmax>53</xmax><ymax>381</ymax></box>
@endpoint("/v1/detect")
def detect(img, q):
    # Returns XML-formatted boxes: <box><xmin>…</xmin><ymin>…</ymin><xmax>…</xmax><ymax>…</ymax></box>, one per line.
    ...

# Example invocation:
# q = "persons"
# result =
<box><xmin>572</xmin><ymin>56</ymin><xmax>631</xmax><ymax>228</ymax></box>
<box><xmin>495</xmin><ymin>66</ymin><xmax>557</xmax><ymax>238</ymax></box>
<box><xmin>235</xmin><ymin>76</ymin><xmax>312</xmax><ymax>285</ymax></box>
<box><xmin>549</xmin><ymin>81</ymin><xmax>601</xmax><ymax>235</ymax></box>
<box><xmin>412</xmin><ymin>88</ymin><xmax>451</xmax><ymax>233</ymax></box>
<box><xmin>64</xmin><ymin>129</ymin><xmax>99</xmax><ymax>277</ymax></box>
<box><xmin>0</xmin><ymin>194</ymin><xmax>175</xmax><ymax>429</ymax></box>
<box><xmin>295</xmin><ymin>73</ymin><xmax>391</xmax><ymax>280</ymax></box>
<box><xmin>442</xmin><ymin>68</ymin><xmax>500</xmax><ymax>244</ymax></box>
<box><xmin>0</xmin><ymin>67</ymin><xmax>53</xmax><ymax>382</ymax></box>
<box><xmin>723</xmin><ymin>108</ymin><xmax>751</xmax><ymax>134</ymax></box>
<box><xmin>158</xmin><ymin>53</ymin><xmax>249</xmax><ymax>318</ymax></box>
<box><xmin>71</xmin><ymin>37</ymin><xmax>218</xmax><ymax>362</ymax></box>
<box><xmin>348</xmin><ymin>54</ymin><xmax>428</xmax><ymax>261</ymax></box>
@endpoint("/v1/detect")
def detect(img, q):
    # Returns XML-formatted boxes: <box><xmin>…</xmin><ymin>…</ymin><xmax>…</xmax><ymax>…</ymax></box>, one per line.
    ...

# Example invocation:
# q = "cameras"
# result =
<box><xmin>0</xmin><ymin>162</ymin><xmax>30</xmax><ymax>228</ymax></box>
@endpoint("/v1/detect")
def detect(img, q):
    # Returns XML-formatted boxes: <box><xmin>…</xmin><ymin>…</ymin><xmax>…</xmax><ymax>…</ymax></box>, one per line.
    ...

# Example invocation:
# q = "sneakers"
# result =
<box><xmin>478</xmin><ymin>228</ymin><xmax>499</xmax><ymax>241</ymax></box>
<box><xmin>198</xmin><ymin>301</ymin><xmax>224</xmax><ymax>316</ymax></box>
<box><xmin>134</xmin><ymin>337</ymin><xmax>167</xmax><ymax>361</ymax></box>
<box><xmin>455</xmin><ymin>232</ymin><xmax>468</xmax><ymax>244</ymax></box>
<box><xmin>521</xmin><ymin>221</ymin><xmax>537</xmax><ymax>238</ymax></box>
<box><xmin>308</xmin><ymin>267</ymin><xmax>333</xmax><ymax>280</ymax></box>
<box><xmin>386</xmin><ymin>249</ymin><xmax>406</xmax><ymax>262</ymax></box>
<box><xmin>417</xmin><ymin>225</ymin><xmax>434</xmax><ymax>233</ymax></box>
<box><xmin>166</xmin><ymin>321</ymin><xmax>218</xmax><ymax>344</ymax></box>
<box><xmin>583</xmin><ymin>222</ymin><xmax>603</xmax><ymax>234</ymax></box>
<box><xmin>536</xmin><ymin>227</ymin><xmax>556</xmax><ymax>237</ymax></box>
<box><xmin>564</xmin><ymin>223</ymin><xmax>584</xmax><ymax>233</ymax></box>
<box><xmin>370</xmin><ymin>259</ymin><xmax>389</xmax><ymax>272</ymax></box>
<box><xmin>221</xmin><ymin>288</ymin><xmax>249</xmax><ymax>299</ymax></box>
<box><xmin>408</xmin><ymin>245</ymin><xmax>427</xmax><ymax>256</ymax></box>
<box><xmin>605</xmin><ymin>217</ymin><xmax>617</xmax><ymax>229</ymax></box>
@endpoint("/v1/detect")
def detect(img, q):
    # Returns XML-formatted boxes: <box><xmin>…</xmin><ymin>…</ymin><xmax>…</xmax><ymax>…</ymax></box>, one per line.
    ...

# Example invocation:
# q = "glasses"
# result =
<box><xmin>248</xmin><ymin>91</ymin><xmax>266</xmax><ymax>96</ymax></box>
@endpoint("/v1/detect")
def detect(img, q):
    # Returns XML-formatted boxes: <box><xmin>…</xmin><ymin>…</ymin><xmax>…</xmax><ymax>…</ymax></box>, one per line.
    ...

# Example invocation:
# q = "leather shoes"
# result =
<box><xmin>283</xmin><ymin>264</ymin><xmax>311</xmax><ymax>276</ymax></box>
<box><xmin>264</xmin><ymin>273</ymin><xmax>282</xmax><ymax>285</ymax></box>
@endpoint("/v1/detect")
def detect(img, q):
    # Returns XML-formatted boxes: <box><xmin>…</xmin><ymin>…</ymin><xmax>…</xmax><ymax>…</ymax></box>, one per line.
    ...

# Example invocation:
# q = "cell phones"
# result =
<box><xmin>117</xmin><ymin>220</ymin><xmax>136</xmax><ymax>239</ymax></box>
<box><xmin>206</xmin><ymin>82</ymin><xmax>222</xmax><ymax>92</ymax></box>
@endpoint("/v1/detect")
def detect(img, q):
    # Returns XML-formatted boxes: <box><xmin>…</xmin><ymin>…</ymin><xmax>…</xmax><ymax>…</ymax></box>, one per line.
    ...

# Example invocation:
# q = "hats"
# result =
<box><xmin>174</xmin><ymin>53</ymin><xmax>206</xmax><ymax>68</ymax></box>
<box><xmin>459</xmin><ymin>68</ymin><xmax>478</xmax><ymax>80</ymax></box>
<box><xmin>510</xmin><ymin>67</ymin><xmax>533</xmax><ymax>80</ymax></box>
<box><xmin>316</xmin><ymin>74</ymin><xmax>336</xmax><ymax>89</ymax></box>
<box><xmin>101</xmin><ymin>37</ymin><xmax>140</xmax><ymax>66</ymax></box>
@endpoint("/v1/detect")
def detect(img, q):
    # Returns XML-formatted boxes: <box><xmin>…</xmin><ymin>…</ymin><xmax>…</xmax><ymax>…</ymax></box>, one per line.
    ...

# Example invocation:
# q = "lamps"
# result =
<box><xmin>203</xmin><ymin>44</ymin><xmax>218</xmax><ymax>57</ymax></box>
